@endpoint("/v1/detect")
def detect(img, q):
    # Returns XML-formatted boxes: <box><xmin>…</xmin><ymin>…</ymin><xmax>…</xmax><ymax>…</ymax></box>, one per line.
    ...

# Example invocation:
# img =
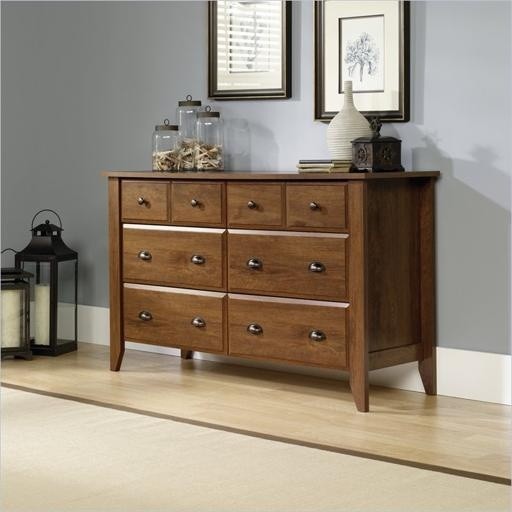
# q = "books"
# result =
<box><xmin>296</xmin><ymin>159</ymin><xmax>352</xmax><ymax>174</ymax></box>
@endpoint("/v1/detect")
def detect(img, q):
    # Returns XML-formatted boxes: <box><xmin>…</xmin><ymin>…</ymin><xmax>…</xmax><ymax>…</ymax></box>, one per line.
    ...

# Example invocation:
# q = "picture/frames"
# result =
<box><xmin>313</xmin><ymin>0</ymin><xmax>410</xmax><ymax>123</ymax></box>
<box><xmin>206</xmin><ymin>0</ymin><xmax>291</xmax><ymax>101</ymax></box>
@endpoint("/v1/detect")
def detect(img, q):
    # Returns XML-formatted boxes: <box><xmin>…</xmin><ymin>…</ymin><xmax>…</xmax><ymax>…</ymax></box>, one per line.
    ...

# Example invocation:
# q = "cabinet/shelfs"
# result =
<box><xmin>99</xmin><ymin>169</ymin><xmax>441</xmax><ymax>413</ymax></box>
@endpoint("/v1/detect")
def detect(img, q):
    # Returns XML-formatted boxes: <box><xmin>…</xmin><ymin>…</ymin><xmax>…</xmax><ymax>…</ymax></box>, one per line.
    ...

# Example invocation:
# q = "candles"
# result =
<box><xmin>1</xmin><ymin>283</ymin><xmax>50</xmax><ymax>348</ymax></box>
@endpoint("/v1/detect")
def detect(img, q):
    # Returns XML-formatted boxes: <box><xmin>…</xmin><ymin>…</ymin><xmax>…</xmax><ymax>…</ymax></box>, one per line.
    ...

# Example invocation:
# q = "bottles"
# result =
<box><xmin>192</xmin><ymin>105</ymin><xmax>227</xmax><ymax>172</ymax></box>
<box><xmin>176</xmin><ymin>94</ymin><xmax>204</xmax><ymax>171</ymax></box>
<box><xmin>151</xmin><ymin>118</ymin><xmax>183</xmax><ymax>175</ymax></box>
